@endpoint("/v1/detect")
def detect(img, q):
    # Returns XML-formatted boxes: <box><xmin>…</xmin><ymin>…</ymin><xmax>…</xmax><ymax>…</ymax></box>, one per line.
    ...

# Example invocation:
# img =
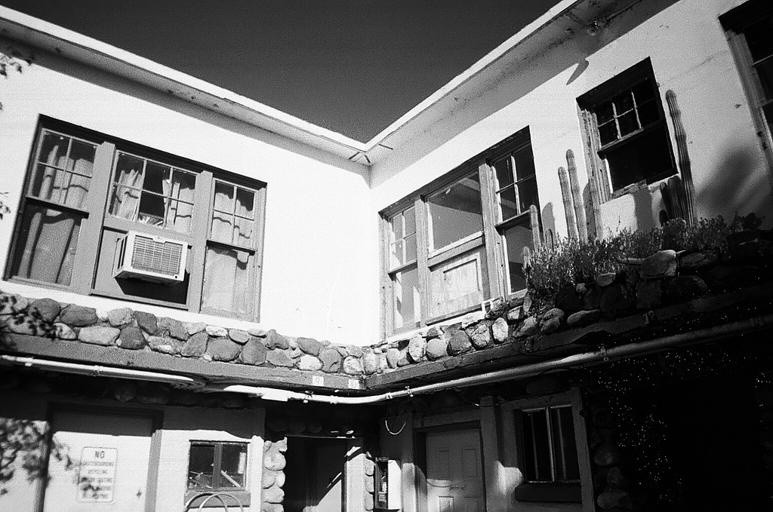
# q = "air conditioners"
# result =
<box><xmin>113</xmin><ymin>230</ymin><xmax>188</xmax><ymax>288</ymax></box>
